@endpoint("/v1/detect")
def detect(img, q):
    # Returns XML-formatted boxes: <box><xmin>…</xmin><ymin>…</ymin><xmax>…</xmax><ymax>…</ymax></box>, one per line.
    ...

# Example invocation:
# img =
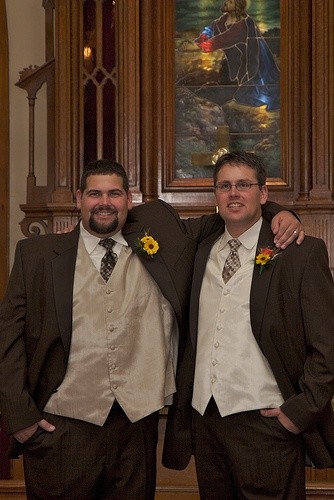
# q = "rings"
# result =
<box><xmin>293</xmin><ymin>231</ymin><xmax>299</xmax><ymax>236</ymax></box>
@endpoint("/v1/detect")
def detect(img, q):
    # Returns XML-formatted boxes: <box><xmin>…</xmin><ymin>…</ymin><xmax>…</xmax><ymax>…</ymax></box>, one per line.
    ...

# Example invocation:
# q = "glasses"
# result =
<box><xmin>215</xmin><ymin>181</ymin><xmax>260</xmax><ymax>192</ymax></box>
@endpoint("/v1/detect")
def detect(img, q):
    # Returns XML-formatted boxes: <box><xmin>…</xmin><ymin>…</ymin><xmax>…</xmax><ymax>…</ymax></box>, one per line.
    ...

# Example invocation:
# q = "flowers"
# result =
<box><xmin>255</xmin><ymin>241</ymin><xmax>281</xmax><ymax>275</ymax></box>
<box><xmin>131</xmin><ymin>228</ymin><xmax>159</xmax><ymax>260</ymax></box>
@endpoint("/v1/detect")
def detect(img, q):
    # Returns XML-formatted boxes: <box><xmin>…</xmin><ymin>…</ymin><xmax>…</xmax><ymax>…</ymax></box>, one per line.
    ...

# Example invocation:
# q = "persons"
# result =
<box><xmin>56</xmin><ymin>151</ymin><xmax>334</xmax><ymax>500</ymax></box>
<box><xmin>0</xmin><ymin>161</ymin><xmax>305</xmax><ymax>500</ymax></box>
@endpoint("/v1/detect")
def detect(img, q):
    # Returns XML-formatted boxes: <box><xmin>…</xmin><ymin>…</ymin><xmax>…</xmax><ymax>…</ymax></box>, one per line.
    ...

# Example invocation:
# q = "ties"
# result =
<box><xmin>98</xmin><ymin>238</ymin><xmax>118</xmax><ymax>283</ymax></box>
<box><xmin>222</xmin><ymin>239</ymin><xmax>242</xmax><ymax>284</ymax></box>
